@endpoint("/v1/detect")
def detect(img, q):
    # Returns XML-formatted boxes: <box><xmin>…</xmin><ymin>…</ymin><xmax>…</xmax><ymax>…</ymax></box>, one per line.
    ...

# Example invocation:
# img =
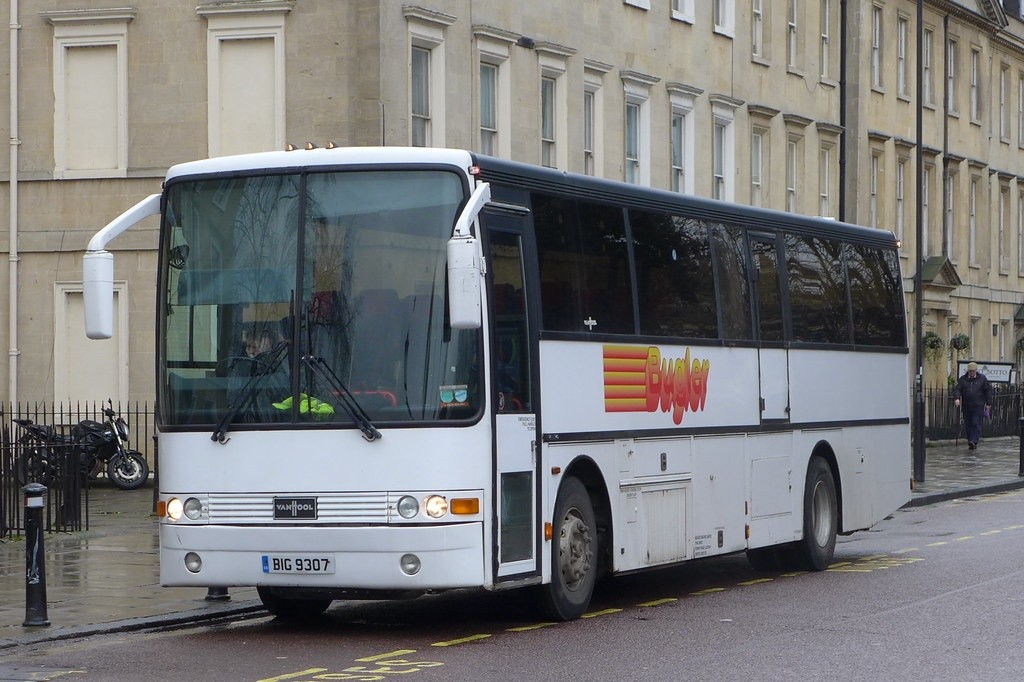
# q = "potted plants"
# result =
<box><xmin>948</xmin><ymin>333</ymin><xmax>972</xmax><ymax>361</ymax></box>
<box><xmin>922</xmin><ymin>332</ymin><xmax>946</xmax><ymax>371</ymax></box>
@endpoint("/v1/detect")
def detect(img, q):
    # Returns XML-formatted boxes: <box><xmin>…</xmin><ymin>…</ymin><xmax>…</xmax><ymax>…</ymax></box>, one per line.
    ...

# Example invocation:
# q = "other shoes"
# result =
<box><xmin>968</xmin><ymin>444</ymin><xmax>977</xmax><ymax>450</ymax></box>
<box><xmin>968</xmin><ymin>441</ymin><xmax>975</xmax><ymax>450</ymax></box>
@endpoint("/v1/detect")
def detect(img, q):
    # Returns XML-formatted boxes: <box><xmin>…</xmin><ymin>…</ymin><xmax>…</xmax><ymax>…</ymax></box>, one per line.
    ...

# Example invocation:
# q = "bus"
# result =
<box><xmin>82</xmin><ymin>143</ymin><xmax>912</xmax><ymax>624</ymax></box>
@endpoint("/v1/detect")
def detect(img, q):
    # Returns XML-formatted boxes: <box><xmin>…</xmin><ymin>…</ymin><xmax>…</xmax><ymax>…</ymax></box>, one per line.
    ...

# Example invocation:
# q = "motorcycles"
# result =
<box><xmin>12</xmin><ymin>397</ymin><xmax>149</xmax><ymax>490</ymax></box>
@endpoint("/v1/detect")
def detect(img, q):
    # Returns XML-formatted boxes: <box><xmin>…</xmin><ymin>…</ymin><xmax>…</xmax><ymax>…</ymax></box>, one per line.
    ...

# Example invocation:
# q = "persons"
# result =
<box><xmin>245</xmin><ymin>322</ymin><xmax>284</xmax><ymax>364</ymax></box>
<box><xmin>954</xmin><ymin>363</ymin><xmax>992</xmax><ymax>450</ymax></box>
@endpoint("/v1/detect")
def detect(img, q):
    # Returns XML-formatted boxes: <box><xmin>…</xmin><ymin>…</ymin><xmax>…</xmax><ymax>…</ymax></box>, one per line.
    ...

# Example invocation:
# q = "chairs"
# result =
<box><xmin>274</xmin><ymin>279</ymin><xmax>587</xmax><ymax>411</ymax></box>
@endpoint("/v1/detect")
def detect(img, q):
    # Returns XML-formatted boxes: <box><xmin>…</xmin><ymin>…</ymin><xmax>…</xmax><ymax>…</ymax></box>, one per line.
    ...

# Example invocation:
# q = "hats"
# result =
<box><xmin>968</xmin><ymin>362</ymin><xmax>978</xmax><ymax>370</ymax></box>
